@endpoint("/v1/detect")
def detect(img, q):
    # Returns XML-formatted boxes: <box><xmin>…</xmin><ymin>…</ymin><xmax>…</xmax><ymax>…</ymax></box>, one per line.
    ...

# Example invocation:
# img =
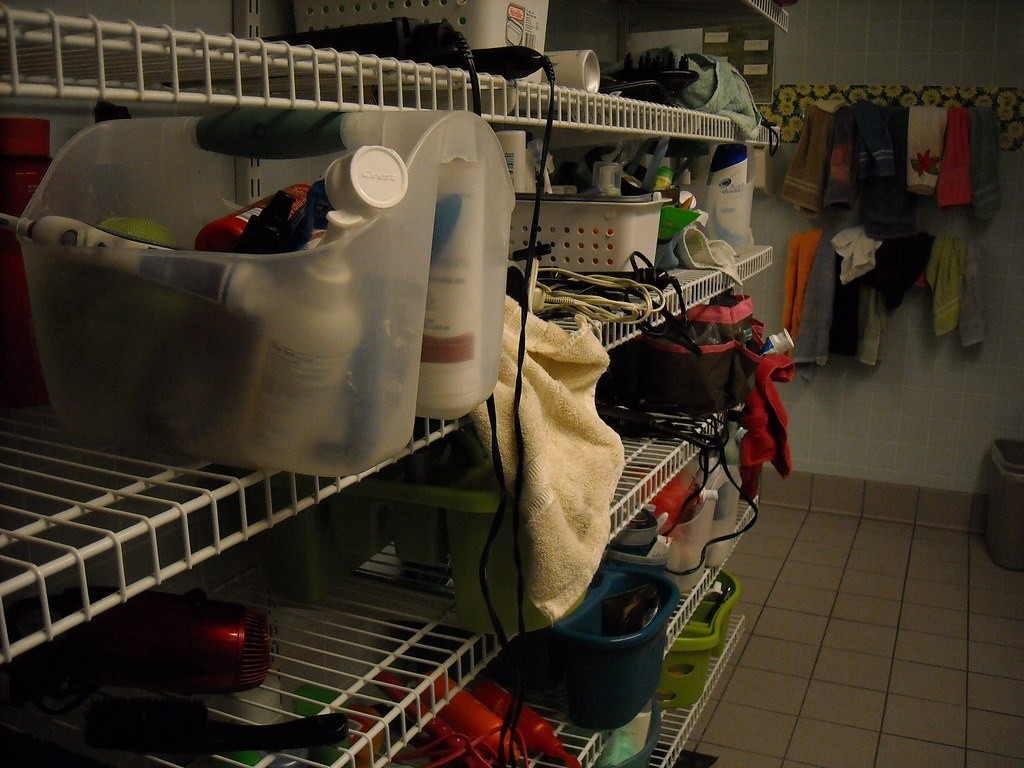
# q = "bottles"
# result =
<box><xmin>650</xmin><ymin>167</ymin><xmax>673</xmax><ymax>190</ymax></box>
<box><xmin>542</xmin><ymin>49</ymin><xmax>600</xmax><ymax>93</ymax></box>
<box><xmin>0</xmin><ymin>117</ymin><xmax>66</xmax><ymax>407</ymax></box>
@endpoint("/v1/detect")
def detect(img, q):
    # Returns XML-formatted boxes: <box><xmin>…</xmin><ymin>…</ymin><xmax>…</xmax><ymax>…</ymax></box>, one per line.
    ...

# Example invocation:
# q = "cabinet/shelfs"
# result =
<box><xmin>0</xmin><ymin>7</ymin><xmax>774</xmax><ymax>768</ymax></box>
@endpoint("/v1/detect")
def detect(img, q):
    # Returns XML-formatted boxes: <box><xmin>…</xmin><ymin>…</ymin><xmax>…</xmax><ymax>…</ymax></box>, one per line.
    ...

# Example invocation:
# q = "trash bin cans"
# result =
<box><xmin>988</xmin><ymin>439</ymin><xmax>1024</xmax><ymax>573</ymax></box>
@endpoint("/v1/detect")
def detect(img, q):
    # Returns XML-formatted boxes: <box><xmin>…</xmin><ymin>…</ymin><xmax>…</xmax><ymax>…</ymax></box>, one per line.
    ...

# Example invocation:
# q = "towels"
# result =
<box><xmin>828</xmin><ymin>224</ymin><xmax>883</xmax><ymax>286</ymax></box>
<box><xmin>736</xmin><ymin>351</ymin><xmax>796</xmax><ymax>501</ymax></box>
<box><xmin>826</xmin><ymin>230</ymin><xmax>937</xmax><ymax>371</ymax></box>
<box><xmin>598</xmin><ymin>43</ymin><xmax>764</xmax><ymax>140</ymax></box>
<box><xmin>925</xmin><ymin>232</ymin><xmax>969</xmax><ymax>337</ymax></box>
<box><xmin>791</xmin><ymin>228</ymin><xmax>838</xmax><ymax>367</ymax></box>
<box><xmin>935</xmin><ymin>107</ymin><xmax>972</xmax><ymax>211</ymax></box>
<box><xmin>778</xmin><ymin>97</ymin><xmax>847</xmax><ymax>221</ymax></box>
<box><xmin>906</xmin><ymin>106</ymin><xmax>948</xmax><ymax>197</ymax></box>
<box><xmin>678</xmin><ymin>223</ymin><xmax>745</xmax><ymax>287</ymax></box>
<box><xmin>957</xmin><ymin>241</ymin><xmax>989</xmax><ymax>349</ymax></box>
<box><xmin>967</xmin><ymin>104</ymin><xmax>1003</xmax><ymax>230</ymax></box>
<box><xmin>449</xmin><ymin>293</ymin><xmax>628</xmax><ymax>624</ymax></box>
<box><xmin>781</xmin><ymin>227</ymin><xmax>824</xmax><ymax>360</ymax></box>
<box><xmin>822</xmin><ymin>99</ymin><xmax>921</xmax><ymax>241</ymax></box>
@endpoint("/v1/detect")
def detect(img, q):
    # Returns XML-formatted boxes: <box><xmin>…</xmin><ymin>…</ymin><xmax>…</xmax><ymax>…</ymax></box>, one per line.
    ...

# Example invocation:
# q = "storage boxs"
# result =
<box><xmin>244</xmin><ymin>468</ymin><xmax>588</xmax><ymax>630</ymax></box>
<box><xmin>656</xmin><ymin>146</ymin><xmax>754</xmax><ymax>255</ymax></box>
<box><xmin>395</xmin><ymin>561</ymin><xmax>679</xmax><ymax>728</ymax></box>
<box><xmin>409</xmin><ymin>112</ymin><xmax>519</xmax><ymax>421</ymax></box>
<box><xmin>16</xmin><ymin>105</ymin><xmax>442</xmax><ymax>474</ymax></box>
<box><xmin>655</xmin><ymin>568</ymin><xmax>739</xmax><ymax>710</ymax></box>
